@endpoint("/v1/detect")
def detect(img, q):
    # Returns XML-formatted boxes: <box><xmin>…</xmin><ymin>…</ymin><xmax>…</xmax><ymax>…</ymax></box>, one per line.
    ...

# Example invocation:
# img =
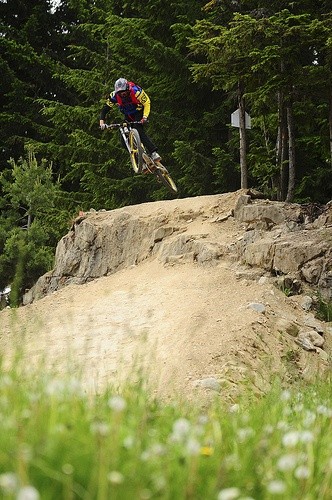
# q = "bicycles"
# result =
<box><xmin>99</xmin><ymin>121</ymin><xmax>178</xmax><ymax>194</ymax></box>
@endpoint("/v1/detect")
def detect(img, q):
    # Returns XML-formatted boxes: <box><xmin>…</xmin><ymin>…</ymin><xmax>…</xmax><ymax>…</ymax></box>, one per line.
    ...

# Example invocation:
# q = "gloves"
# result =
<box><xmin>101</xmin><ymin>124</ymin><xmax>108</xmax><ymax>130</ymax></box>
<box><xmin>141</xmin><ymin>117</ymin><xmax>148</xmax><ymax>125</ymax></box>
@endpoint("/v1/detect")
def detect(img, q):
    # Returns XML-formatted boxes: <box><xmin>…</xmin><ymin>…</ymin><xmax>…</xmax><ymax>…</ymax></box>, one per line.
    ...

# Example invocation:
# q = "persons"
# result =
<box><xmin>100</xmin><ymin>78</ymin><xmax>161</xmax><ymax>171</ymax></box>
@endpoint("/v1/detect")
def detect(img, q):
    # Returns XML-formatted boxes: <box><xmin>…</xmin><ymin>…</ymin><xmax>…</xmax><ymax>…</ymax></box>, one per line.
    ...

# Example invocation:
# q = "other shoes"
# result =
<box><xmin>151</xmin><ymin>152</ymin><xmax>162</xmax><ymax>161</ymax></box>
<box><xmin>141</xmin><ymin>163</ymin><xmax>147</xmax><ymax>171</ymax></box>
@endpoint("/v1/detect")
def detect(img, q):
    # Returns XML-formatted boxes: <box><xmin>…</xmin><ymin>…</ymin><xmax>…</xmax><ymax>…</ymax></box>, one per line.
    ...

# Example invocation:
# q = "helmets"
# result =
<box><xmin>114</xmin><ymin>78</ymin><xmax>129</xmax><ymax>96</ymax></box>
